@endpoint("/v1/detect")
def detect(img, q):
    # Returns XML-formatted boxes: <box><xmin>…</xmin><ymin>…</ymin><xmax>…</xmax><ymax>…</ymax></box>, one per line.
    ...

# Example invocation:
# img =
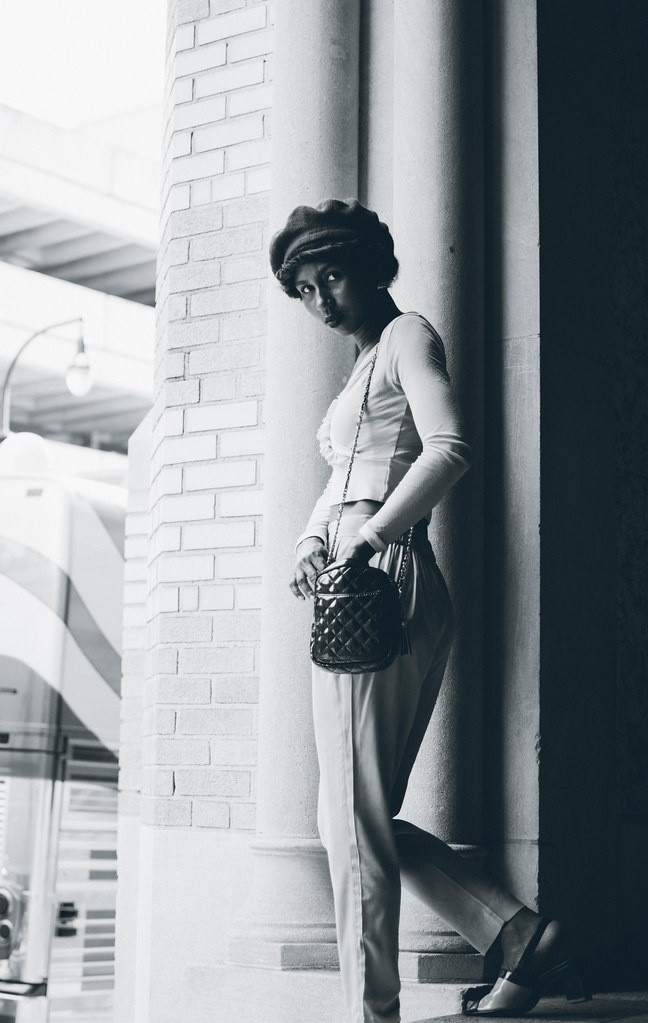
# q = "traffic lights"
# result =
<box><xmin>0</xmin><ymin>882</ymin><xmax>21</xmax><ymax>960</ymax></box>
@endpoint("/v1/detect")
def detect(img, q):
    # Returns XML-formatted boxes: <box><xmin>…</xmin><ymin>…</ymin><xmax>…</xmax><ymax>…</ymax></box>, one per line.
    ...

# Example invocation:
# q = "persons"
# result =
<box><xmin>267</xmin><ymin>198</ymin><xmax>594</xmax><ymax>1023</ymax></box>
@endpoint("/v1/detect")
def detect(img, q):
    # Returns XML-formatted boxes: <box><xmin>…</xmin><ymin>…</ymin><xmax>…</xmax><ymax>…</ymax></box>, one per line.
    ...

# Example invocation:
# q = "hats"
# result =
<box><xmin>269</xmin><ymin>199</ymin><xmax>399</xmax><ymax>298</ymax></box>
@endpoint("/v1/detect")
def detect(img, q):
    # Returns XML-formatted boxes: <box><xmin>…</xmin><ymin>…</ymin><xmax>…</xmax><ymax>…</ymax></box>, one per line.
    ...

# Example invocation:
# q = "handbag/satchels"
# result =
<box><xmin>309</xmin><ymin>558</ymin><xmax>411</xmax><ymax>674</ymax></box>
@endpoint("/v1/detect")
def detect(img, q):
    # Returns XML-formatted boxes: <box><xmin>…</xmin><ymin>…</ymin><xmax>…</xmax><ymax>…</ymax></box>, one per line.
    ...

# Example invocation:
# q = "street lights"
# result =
<box><xmin>0</xmin><ymin>314</ymin><xmax>91</xmax><ymax>440</ymax></box>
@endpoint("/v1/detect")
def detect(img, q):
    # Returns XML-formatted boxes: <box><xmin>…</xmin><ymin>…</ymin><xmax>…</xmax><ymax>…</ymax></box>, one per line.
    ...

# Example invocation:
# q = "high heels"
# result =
<box><xmin>461</xmin><ymin>918</ymin><xmax>593</xmax><ymax>1016</ymax></box>
